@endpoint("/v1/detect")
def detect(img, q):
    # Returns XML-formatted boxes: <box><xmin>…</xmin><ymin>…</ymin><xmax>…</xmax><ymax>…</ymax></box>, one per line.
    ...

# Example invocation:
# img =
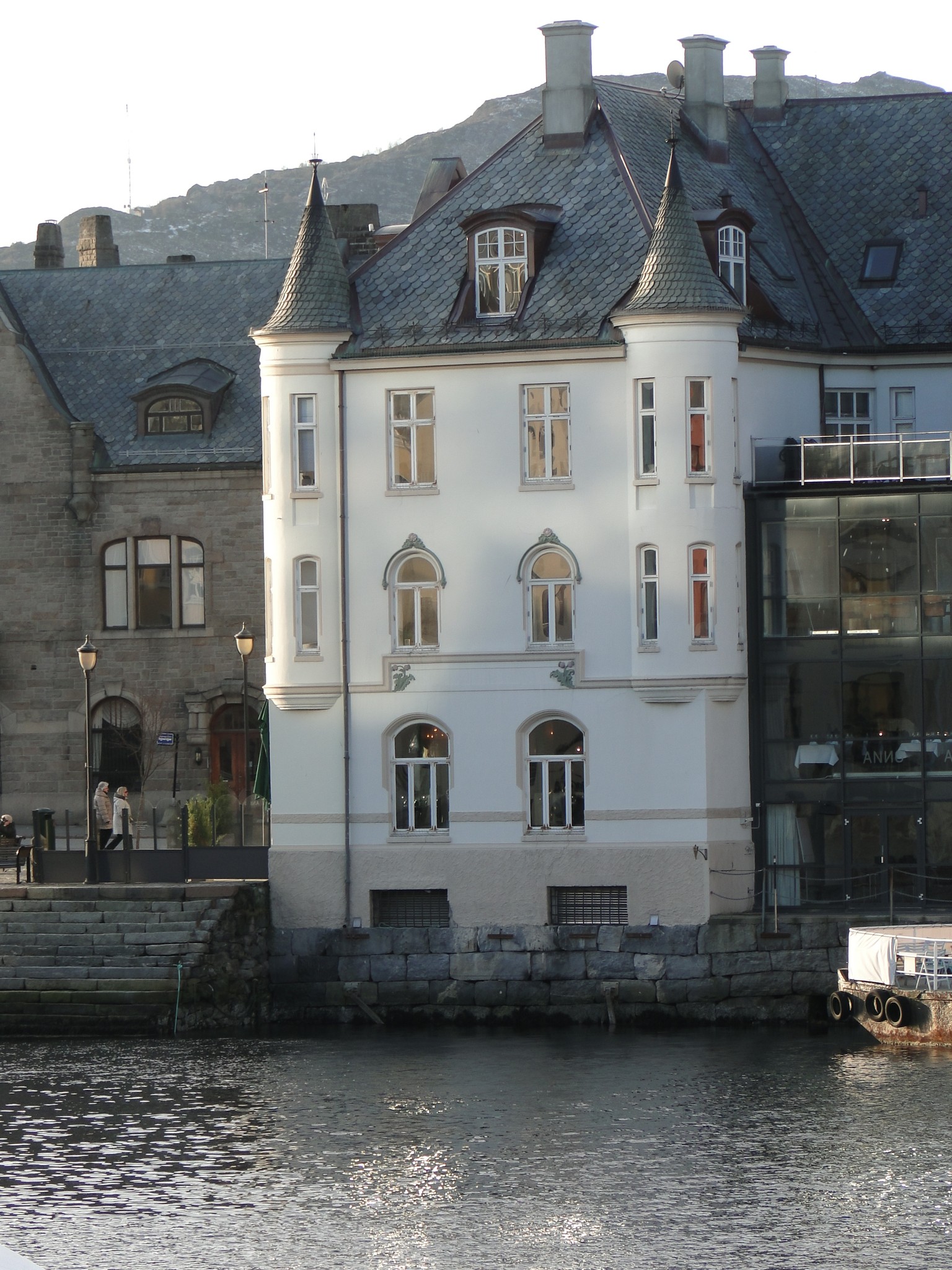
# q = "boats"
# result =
<box><xmin>829</xmin><ymin>924</ymin><xmax>952</xmax><ymax>1052</ymax></box>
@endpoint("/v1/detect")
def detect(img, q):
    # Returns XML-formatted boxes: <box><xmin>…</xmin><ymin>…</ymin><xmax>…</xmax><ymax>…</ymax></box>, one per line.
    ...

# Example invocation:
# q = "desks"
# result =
<box><xmin>896</xmin><ymin>742</ymin><xmax>949</xmax><ymax>770</ymax></box>
<box><xmin>795</xmin><ymin>745</ymin><xmax>838</xmax><ymax>777</ymax></box>
<box><xmin>826</xmin><ymin>741</ymin><xmax>868</xmax><ymax>762</ymax></box>
<box><xmin>901</xmin><ymin>453</ymin><xmax>949</xmax><ymax>483</ymax></box>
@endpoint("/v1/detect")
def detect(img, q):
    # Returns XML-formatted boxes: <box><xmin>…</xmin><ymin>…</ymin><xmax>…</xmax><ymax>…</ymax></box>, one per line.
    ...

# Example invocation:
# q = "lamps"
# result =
<box><xmin>195</xmin><ymin>747</ymin><xmax>202</xmax><ymax>766</ymax></box>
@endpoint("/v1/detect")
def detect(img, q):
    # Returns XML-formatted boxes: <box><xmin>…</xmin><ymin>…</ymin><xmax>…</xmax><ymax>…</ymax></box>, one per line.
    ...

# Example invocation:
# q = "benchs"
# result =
<box><xmin>0</xmin><ymin>835</ymin><xmax>34</xmax><ymax>885</ymax></box>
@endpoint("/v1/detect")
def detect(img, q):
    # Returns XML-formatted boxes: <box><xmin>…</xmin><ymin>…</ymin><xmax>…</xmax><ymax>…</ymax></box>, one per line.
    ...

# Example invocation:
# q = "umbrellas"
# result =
<box><xmin>254</xmin><ymin>699</ymin><xmax>271</xmax><ymax>845</ymax></box>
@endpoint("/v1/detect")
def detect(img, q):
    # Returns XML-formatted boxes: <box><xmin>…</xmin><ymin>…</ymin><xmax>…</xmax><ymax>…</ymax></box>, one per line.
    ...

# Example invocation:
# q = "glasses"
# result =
<box><xmin>125</xmin><ymin>791</ymin><xmax>128</xmax><ymax>793</ymax></box>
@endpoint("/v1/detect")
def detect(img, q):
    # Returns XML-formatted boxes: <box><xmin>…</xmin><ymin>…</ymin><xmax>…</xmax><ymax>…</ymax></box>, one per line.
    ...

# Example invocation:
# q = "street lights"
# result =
<box><xmin>76</xmin><ymin>635</ymin><xmax>100</xmax><ymax>885</ymax></box>
<box><xmin>233</xmin><ymin>621</ymin><xmax>255</xmax><ymax>798</ymax></box>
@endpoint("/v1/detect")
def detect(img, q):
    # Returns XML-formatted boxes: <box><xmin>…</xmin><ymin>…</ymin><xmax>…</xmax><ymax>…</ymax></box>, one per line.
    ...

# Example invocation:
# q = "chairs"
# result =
<box><xmin>840</xmin><ymin>592</ymin><xmax>952</xmax><ymax>635</ymax></box>
<box><xmin>824</xmin><ymin>737</ymin><xmax>952</xmax><ymax>768</ymax></box>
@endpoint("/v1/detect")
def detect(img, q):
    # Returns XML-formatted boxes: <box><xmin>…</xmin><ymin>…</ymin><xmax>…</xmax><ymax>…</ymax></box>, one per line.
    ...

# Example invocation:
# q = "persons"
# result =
<box><xmin>104</xmin><ymin>787</ymin><xmax>134</xmax><ymax>851</ymax></box>
<box><xmin>0</xmin><ymin>814</ymin><xmax>18</xmax><ymax>840</ymax></box>
<box><xmin>93</xmin><ymin>782</ymin><xmax>113</xmax><ymax>850</ymax></box>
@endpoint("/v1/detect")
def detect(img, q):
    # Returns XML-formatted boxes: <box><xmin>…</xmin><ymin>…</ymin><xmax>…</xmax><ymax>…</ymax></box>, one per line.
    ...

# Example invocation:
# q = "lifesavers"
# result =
<box><xmin>865</xmin><ymin>989</ymin><xmax>888</xmax><ymax>1022</ymax></box>
<box><xmin>829</xmin><ymin>991</ymin><xmax>851</xmax><ymax>1022</ymax></box>
<box><xmin>885</xmin><ymin>995</ymin><xmax>912</xmax><ymax>1028</ymax></box>
